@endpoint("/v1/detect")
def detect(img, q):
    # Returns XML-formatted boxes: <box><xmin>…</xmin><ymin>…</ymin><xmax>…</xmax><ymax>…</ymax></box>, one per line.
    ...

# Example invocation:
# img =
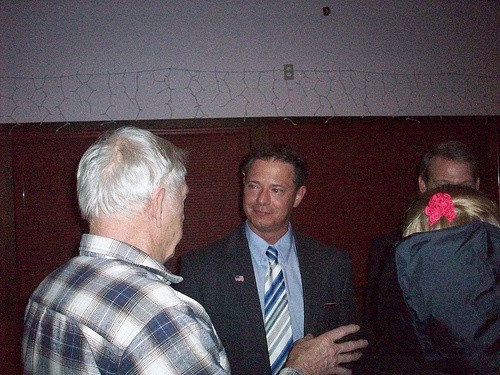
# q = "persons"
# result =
<box><xmin>177</xmin><ymin>142</ymin><xmax>353</xmax><ymax>375</ymax></box>
<box><xmin>367</xmin><ymin>139</ymin><xmax>500</xmax><ymax>375</ymax></box>
<box><xmin>22</xmin><ymin>126</ymin><xmax>368</xmax><ymax>375</ymax></box>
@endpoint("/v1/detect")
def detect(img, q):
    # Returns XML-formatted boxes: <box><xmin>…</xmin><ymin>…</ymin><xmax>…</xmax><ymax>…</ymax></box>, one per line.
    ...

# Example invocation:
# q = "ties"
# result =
<box><xmin>263</xmin><ymin>245</ymin><xmax>294</xmax><ymax>375</ymax></box>
